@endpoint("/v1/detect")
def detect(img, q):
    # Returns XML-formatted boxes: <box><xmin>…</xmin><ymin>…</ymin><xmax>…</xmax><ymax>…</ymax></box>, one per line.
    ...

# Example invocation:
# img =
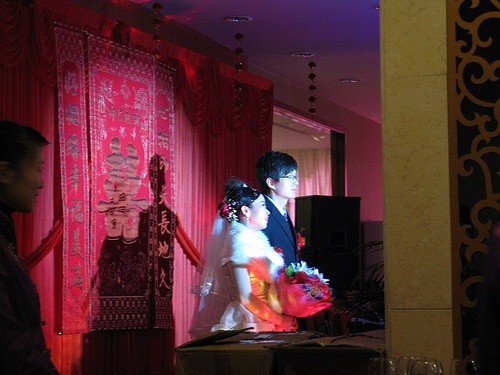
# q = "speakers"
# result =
<box><xmin>294</xmin><ymin>195</ymin><xmax>362</xmax><ymax>303</ymax></box>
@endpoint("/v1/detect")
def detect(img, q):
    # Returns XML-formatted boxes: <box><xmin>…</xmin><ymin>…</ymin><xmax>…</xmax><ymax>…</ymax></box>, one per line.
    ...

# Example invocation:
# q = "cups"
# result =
<box><xmin>368</xmin><ymin>355</ymin><xmax>479</xmax><ymax>375</ymax></box>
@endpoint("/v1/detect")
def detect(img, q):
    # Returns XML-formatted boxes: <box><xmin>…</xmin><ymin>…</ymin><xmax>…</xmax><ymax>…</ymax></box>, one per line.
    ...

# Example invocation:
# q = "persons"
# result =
<box><xmin>255</xmin><ymin>150</ymin><xmax>300</xmax><ymax>270</ymax></box>
<box><xmin>211</xmin><ymin>180</ymin><xmax>299</xmax><ymax>333</ymax></box>
<box><xmin>0</xmin><ymin>120</ymin><xmax>68</xmax><ymax>375</ymax></box>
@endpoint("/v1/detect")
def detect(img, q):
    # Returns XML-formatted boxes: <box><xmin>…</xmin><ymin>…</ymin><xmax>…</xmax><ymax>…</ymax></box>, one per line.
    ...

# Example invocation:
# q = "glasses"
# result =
<box><xmin>279</xmin><ymin>175</ymin><xmax>298</xmax><ymax>181</ymax></box>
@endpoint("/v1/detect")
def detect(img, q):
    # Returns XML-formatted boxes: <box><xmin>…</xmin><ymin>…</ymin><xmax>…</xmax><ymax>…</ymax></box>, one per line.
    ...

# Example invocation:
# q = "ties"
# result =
<box><xmin>283</xmin><ymin>209</ymin><xmax>288</xmax><ymax>222</ymax></box>
